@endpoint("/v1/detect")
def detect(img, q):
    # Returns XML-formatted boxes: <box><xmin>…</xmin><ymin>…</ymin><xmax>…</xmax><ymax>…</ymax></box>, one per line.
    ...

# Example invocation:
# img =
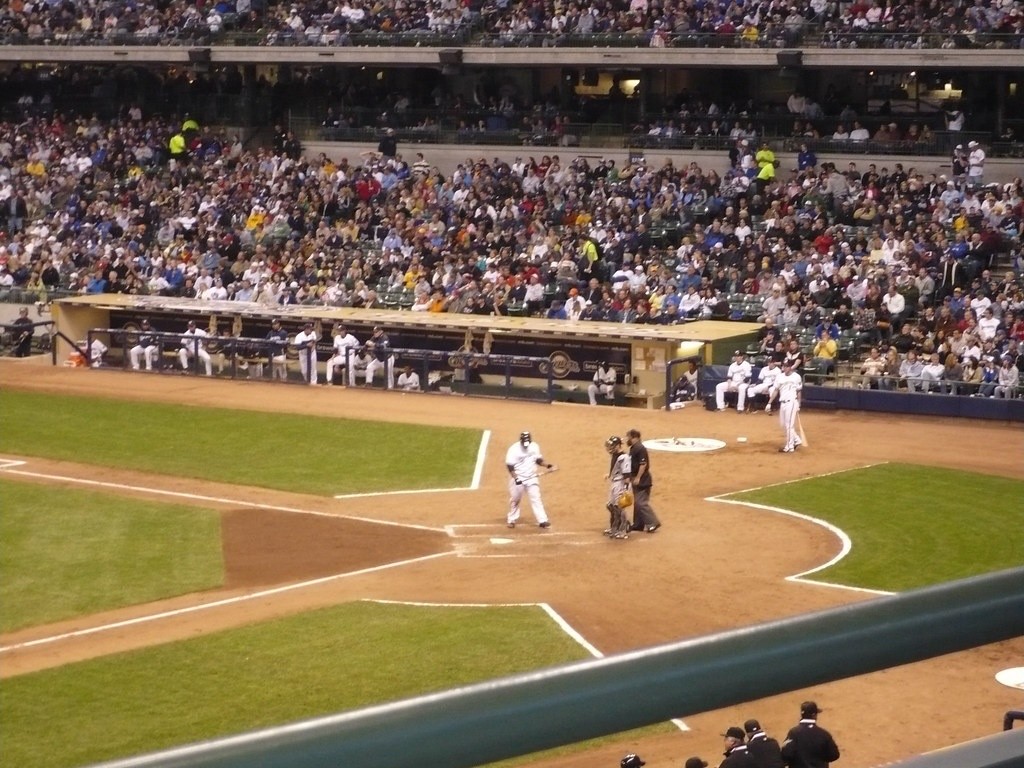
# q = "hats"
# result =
<box><xmin>801</xmin><ymin>701</ymin><xmax>823</xmax><ymax>715</ymax></box>
<box><xmin>734</xmin><ymin>349</ymin><xmax>743</xmax><ymax>355</ymax></box>
<box><xmin>685</xmin><ymin>757</ymin><xmax>708</xmax><ymax>768</ymax></box>
<box><xmin>720</xmin><ymin>727</ymin><xmax>745</xmax><ymax>740</ymax></box>
<box><xmin>744</xmin><ymin>719</ymin><xmax>762</xmax><ymax>731</ymax></box>
<box><xmin>767</xmin><ymin>355</ymin><xmax>775</xmax><ymax>363</ymax></box>
<box><xmin>783</xmin><ymin>358</ymin><xmax>791</xmax><ymax>366</ymax></box>
<box><xmin>622</xmin><ymin>754</ymin><xmax>646</xmax><ymax>768</ymax></box>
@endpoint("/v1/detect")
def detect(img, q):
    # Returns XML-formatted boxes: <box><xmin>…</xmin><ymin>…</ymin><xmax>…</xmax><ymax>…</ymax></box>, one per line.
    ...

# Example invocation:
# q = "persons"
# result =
<box><xmin>621</xmin><ymin>701</ymin><xmax>840</xmax><ymax>768</ymax></box>
<box><xmin>0</xmin><ymin>0</ymin><xmax>1024</xmax><ymax>538</ymax></box>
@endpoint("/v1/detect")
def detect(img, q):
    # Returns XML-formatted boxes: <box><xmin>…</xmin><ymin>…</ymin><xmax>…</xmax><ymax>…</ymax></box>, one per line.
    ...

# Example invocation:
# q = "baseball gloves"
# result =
<box><xmin>618</xmin><ymin>491</ymin><xmax>633</xmax><ymax>509</ymax></box>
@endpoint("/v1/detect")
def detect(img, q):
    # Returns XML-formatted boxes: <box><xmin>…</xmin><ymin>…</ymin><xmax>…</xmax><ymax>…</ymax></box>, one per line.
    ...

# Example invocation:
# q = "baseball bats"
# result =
<box><xmin>596</xmin><ymin>360</ymin><xmax>600</xmax><ymax>391</ymax></box>
<box><xmin>797</xmin><ymin>412</ymin><xmax>808</xmax><ymax>447</ymax></box>
<box><xmin>521</xmin><ymin>465</ymin><xmax>560</xmax><ymax>482</ymax></box>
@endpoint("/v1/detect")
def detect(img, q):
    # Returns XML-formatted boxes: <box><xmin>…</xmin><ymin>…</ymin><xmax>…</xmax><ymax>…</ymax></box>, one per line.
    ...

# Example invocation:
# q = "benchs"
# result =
<box><xmin>551</xmin><ymin>387</ymin><xmax>630</xmax><ymax>406</ymax></box>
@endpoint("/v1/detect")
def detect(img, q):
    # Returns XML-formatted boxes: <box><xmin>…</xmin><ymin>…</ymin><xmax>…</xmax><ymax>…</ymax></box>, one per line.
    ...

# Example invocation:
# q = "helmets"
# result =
<box><xmin>374</xmin><ymin>327</ymin><xmax>381</xmax><ymax>333</ymax></box>
<box><xmin>188</xmin><ymin>321</ymin><xmax>196</xmax><ymax>327</ymax></box>
<box><xmin>605</xmin><ymin>436</ymin><xmax>621</xmax><ymax>448</ymax></box>
<box><xmin>141</xmin><ymin>319</ymin><xmax>150</xmax><ymax>325</ymax></box>
<box><xmin>306</xmin><ymin>323</ymin><xmax>314</xmax><ymax>328</ymax></box>
<box><xmin>271</xmin><ymin>319</ymin><xmax>280</xmax><ymax>325</ymax></box>
<box><xmin>748</xmin><ymin>345</ymin><xmax>758</xmax><ymax>354</ymax></box>
<box><xmin>339</xmin><ymin>325</ymin><xmax>346</xmax><ymax>331</ymax></box>
<box><xmin>520</xmin><ymin>432</ymin><xmax>531</xmax><ymax>448</ymax></box>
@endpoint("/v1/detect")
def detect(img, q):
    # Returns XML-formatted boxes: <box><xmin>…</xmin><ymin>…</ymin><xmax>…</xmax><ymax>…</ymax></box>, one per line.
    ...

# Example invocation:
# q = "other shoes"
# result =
<box><xmin>647</xmin><ymin>524</ymin><xmax>661</xmax><ymax>533</ymax></box>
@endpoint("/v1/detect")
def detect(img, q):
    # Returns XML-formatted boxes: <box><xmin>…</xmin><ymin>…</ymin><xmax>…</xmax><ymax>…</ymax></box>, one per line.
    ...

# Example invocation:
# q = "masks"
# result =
<box><xmin>627</xmin><ymin>440</ymin><xmax>633</xmax><ymax>447</ymax></box>
